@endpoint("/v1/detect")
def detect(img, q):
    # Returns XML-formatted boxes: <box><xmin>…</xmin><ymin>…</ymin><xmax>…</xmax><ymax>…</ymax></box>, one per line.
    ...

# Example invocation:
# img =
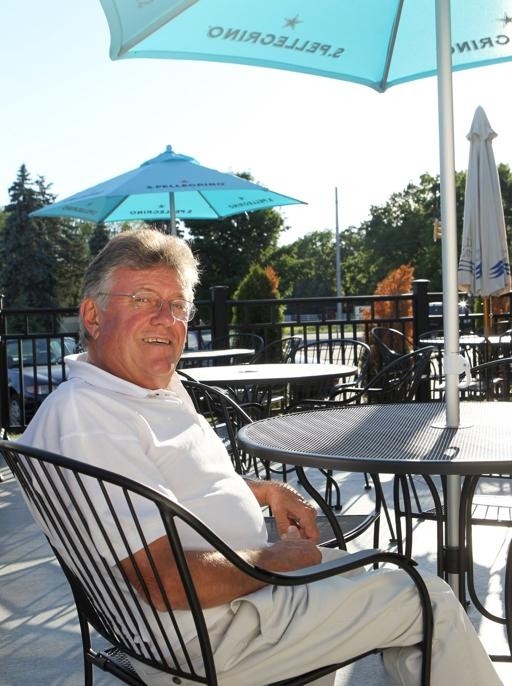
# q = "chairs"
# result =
<box><xmin>172</xmin><ymin>326</ymin><xmax>382</xmax><ymax>551</ymax></box>
<box><xmin>370</xmin><ymin>326</ymin><xmax>511</xmax><ymax>403</ymax></box>
<box><xmin>1</xmin><ymin>439</ymin><xmax>433</xmax><ymax>686</ymax></box>
<box><xmin>0</xmin><ymin>324</ymin><xmax>83</xmax><ymax>436</ymax></box>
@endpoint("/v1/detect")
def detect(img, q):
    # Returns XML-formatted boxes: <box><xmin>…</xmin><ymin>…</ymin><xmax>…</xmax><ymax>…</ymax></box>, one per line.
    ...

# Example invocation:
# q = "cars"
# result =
<box><xmin>1</xmin><ymin>334</ymin><xmax>86</xmax><ymax>435</ymax></box>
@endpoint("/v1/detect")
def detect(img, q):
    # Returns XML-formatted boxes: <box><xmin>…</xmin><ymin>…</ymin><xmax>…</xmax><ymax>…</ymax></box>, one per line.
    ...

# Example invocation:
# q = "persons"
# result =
<box><xmin>14</xmin><ymin>226</ymin><xmax>505</xmax><ymax>686</ymax></box>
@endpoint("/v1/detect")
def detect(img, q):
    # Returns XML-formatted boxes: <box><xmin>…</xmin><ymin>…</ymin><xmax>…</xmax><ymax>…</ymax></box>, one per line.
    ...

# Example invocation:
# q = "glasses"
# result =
<box><xmin>90</xmin><ymin>288</ymin><xmax>201</xmax><ymax>323</ymax></box>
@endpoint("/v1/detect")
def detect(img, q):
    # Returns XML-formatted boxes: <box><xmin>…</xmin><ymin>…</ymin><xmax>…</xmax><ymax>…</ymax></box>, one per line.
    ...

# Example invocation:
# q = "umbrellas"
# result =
<box><xmin>98</xmin><ymin>0</ymin><xmax>511</xmax><ymax>602</ymax></box>
<box><xmin>457</xmin><ymin>105</ymin><xmax>512</xmax><ymax>402</ymax></box>
<box><xmin>26</xmin><ymin>143</ymin><xmax>309</xmax><ymax>237</ymax></box>
